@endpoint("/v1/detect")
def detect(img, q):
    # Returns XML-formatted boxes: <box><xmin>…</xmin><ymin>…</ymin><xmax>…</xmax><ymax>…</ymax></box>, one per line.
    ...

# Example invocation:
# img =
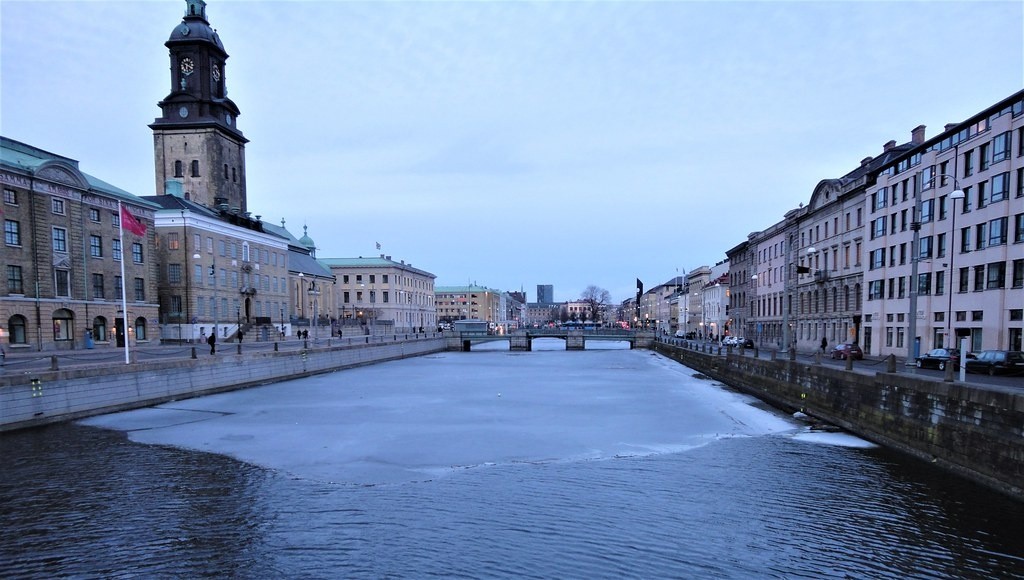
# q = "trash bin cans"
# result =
<box><xmin>0</xmin><ymin>349</ymin><xmax>5</xmax><ymax>366</ymax></box>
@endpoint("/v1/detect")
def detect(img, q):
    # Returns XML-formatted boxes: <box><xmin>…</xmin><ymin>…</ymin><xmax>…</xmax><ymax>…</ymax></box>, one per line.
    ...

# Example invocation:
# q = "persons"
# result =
<box><xmin>710</xmin><ymin>333</ymin><xmax>712</xmax><ymax>340</ymax></box>
<box><xmin>208</xmin><ymin>333</ymin><xmax>216</xmax><ymax>355</ymax></box>
<box><xmin>822</xmin><ymin>337</ymin><xmax>827</xmax><ymax>353</ymax></box>
<box><xmin>338</xmin><ymin>330</ymin><xmax>342</xmax><ymax>338</ymax></box>
<box><xmin>238</xmin><ymin>330</ymin><xmax>243</xmax><ymax>344</ymax></box>
<box><xmin>691</xmin><ymin>330</ymin><xmax>696</xmax><ymax>338</ymax></box>
<box><xmin>297</xmin><ymin>329</ymin><xmax>307</xmax><ymax>340</ymax></box>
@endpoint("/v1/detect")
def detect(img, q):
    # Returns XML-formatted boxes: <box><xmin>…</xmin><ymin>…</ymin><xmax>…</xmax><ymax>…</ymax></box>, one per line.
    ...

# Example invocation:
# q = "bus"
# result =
<box><xmin>559</xmin><ymin>322</ymin><xmax>603</xmax><ymax>331</ymax></box>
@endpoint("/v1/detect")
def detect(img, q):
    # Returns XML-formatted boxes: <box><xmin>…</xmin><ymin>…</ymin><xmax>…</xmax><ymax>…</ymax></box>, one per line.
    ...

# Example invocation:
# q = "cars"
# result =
<box><xmin>914</xmin><ymin>348</ymin><xmax>975</xmax><ymax>371</ymax></box>
<box><xmin>966</xmin><ymin>349</ymin><xmax>1024</xmax><ymax>376</ymax></box>
<box><xmin>674</xmin><ymin>330</ymin><xmax>685</xmax><ymax>338</ymax></box>
<box><xmin>830</xmin><ymin>344</ymin><xmax>863</xmax><ymax>360</ymax></box>
<box><xmin>722</xmin><ymin>336</ymin><xmax>754</xmax><ymax>349</ymax></box>
<box><xmin>438</xmin><ymin>323</ymin><xmax>455</xmax><ymax>332</ymax></box>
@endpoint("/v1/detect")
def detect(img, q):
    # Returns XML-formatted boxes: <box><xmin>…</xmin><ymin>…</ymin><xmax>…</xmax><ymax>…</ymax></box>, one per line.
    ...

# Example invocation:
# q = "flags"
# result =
<box><xmin>121</xmin><ymin>204</ymin><xmax>147</xmax><ymax>237</ymax></box>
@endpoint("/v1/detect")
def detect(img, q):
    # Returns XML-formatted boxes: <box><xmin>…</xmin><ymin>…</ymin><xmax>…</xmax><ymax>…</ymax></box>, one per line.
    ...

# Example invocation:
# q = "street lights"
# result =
<box><xmin>342</xmin><ymin>305</ymin><xmax>346</xmax><ymax>325</ymax></box>
<box><xmin>904</xmin><ymin>170</ymin><xmax>965</xmax><ymax>368</ymax></box>
<box><xmin>280</xmin><ymin>308</ymin><xmax>286</xmax><ymax>337</ymax></box>
<box><xmin>235</xmin><ymin>305</ymin><xmax>242</xmax><ymax>344</ymax></box>
